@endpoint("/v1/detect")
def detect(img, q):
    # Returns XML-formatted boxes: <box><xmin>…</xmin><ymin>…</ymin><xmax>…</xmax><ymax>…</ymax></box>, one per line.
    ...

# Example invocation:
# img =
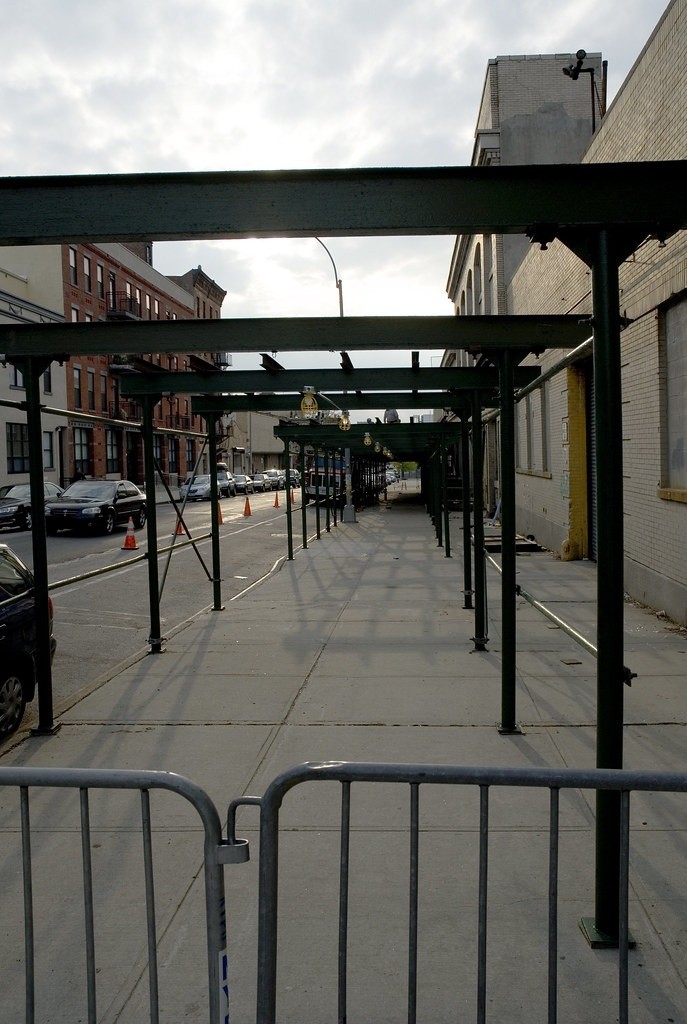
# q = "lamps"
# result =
<box><xmin>338</xmin><ymin>411</ymin><xmax>351</xmax><ymax>430</ymax></box>
<box><xmin>298</xmin><ymin>385</ymin><xmax>318</xmax><ymax>419</ymax></box>
<box><xmin>363</xmin><ymin>432</ymin><xmax>392</xmax><ymax>457</ymax></box>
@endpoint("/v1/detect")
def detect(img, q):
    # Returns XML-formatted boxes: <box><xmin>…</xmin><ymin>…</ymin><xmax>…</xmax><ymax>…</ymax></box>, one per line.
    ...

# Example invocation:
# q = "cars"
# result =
<box><xmin>261</xmin><ymin>470</ymin><xmax>286</xmax><ymax>490</ymax></box>
<box><xmin>249</xmin><ymin>474</ymin><xmax>273</xmax><ymax>492</ymax></box>
<box><xmin>366</xmin><ymin>465</ymin><xmax>398</xmax><ymax>488</ymax></box>
<box><xmin>235</xmin><ymin>474</ymin><xmax>254</xmax><ymax>495</ymax></box>
<box><xmin>45</xmin><ymin>478</ymin><xmax>147</xmax><ymax>537</ymax></box>
<box><xmin>217</xmin><ymin>472</ymin><xmax>237</xmax><ymax>497</ymax></box>
<box><xmin>179</xmin><ymin>475</ymin><xmax>222</xmax><ymax>503</ymax></box>
<box><xmin>287</xmin><ymin>468</ymin><xmax>301</xmax><ymax>489</ymax></box>
<box><xmin>0</xmin><ymin>542</ymin><xmax>57</xmax><ymax>745</ymax></box>
<box><xmin>0</xmin><ymin>482</ymin><xmax>65</xmax><ymax>532</ymax></box>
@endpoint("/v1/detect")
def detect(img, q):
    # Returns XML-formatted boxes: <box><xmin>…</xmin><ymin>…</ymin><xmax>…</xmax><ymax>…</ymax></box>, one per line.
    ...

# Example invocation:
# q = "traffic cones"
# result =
<box><xmin>171</xmin><ymin>509</ymin><xmax>185</xmax><ymax>535</ymax></box>
<box><xmin>218</xmin><ymin>501</ymin><xmax>225</xmax><ymax>525</ymax></box>
<box><xmin>291</xmin><ymin>490</ymin><xmax>296</xmax><ymax>505</ymax></box>
<box><xmin>242</xmin><ymin>496</ymin><xmax>253</xmax><ymax>517</ymax></box>
<box><xmin>120</xmin><ymin>517</ymin><xmax>140</xmax><ymax>550</ymax></box>
<box><xmin>273</xmin><ymin>492</ymin><xmax>281</xmax><ymax>507</ymax></box>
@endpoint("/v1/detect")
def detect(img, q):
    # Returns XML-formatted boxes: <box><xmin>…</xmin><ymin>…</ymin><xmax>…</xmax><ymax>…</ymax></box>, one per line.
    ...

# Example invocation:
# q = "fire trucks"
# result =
<box><xmin>300</xmin><ymin>448</ymin><xmax>364</xmax><ymax>508</ymax></box>
